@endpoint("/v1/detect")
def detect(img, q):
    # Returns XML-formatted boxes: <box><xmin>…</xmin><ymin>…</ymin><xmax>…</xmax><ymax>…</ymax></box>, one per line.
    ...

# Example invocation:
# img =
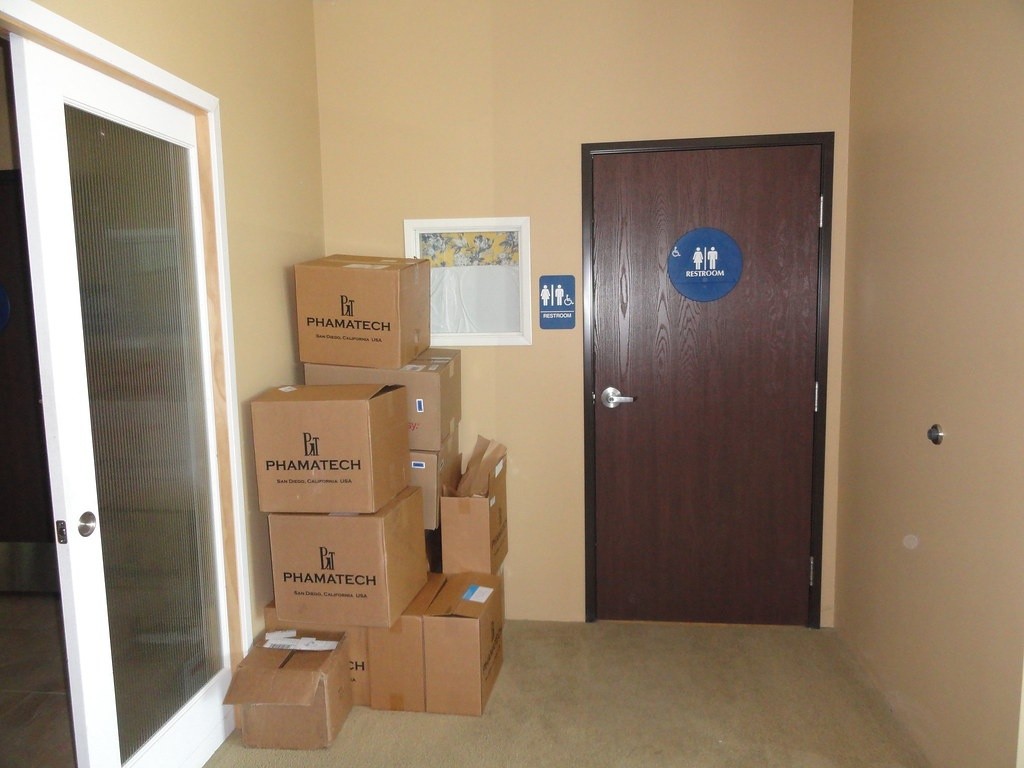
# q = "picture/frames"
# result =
<box><xmin>403</xmin><ymin>217</ymin><xmax>532</xmax><ymax>346</ymax></box>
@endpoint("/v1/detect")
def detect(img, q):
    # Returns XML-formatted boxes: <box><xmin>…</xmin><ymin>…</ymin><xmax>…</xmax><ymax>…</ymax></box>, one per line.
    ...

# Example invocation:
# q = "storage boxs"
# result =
<box><xmin>222</xmin><ymin>254</ymin><xmax>509</xmax><ymax>752</ymax></box>
<box><xmin>92</xmin><ymin>386</ymin><xmax>214</xmax><ymax>702</ymax></box>
